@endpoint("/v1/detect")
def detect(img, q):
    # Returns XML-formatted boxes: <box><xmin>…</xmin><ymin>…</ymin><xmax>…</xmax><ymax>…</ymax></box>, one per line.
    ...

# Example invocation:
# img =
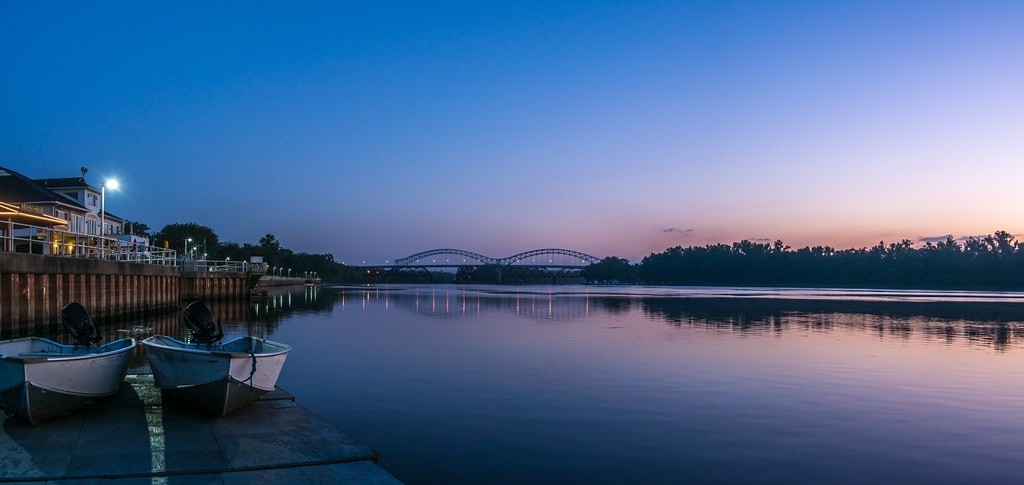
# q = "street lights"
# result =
<box><xmin>225</xmin><ymin>258</ymin><xmax>230</xmax><ymax>271</ymax></box>
<box><xmin>185</xmin><ymin>239</ymin><xmax>192</xmax><ymax>255</ymax></box>
<box><xmin>280</xmin><ymin>268</ymin><xmax>283</xmax><ymax>279</ymax></box>
<box><xmin>288</xmin><ymin>269</ymin><xmax>291</xmax><ymax>278</ymax></box>
<box><xmin>273</xmin><ymin>267</ymin><xmax>276</xmax><ymax>279</ymax></box>
<box><xmin>100</xmin><ymin>178</ymin><xmax>118</xmax><ymax>258</ymax></box>
<box><xmin>305</xmin><ymin>271</ymin><xmax>316</xmax><ymax>278</ymax></box>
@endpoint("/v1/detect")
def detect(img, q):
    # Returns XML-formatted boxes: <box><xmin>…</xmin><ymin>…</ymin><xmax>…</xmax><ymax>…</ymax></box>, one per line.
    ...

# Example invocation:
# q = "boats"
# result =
<box><xmin>0</xmin><ymin>301</ymin><xmax>137</xmax><ymax>427</ymax></box>
<box><xmin>140</xmin><ymin>299</ymin><xmax>292</xmax><ymax>415</ymax></box>
